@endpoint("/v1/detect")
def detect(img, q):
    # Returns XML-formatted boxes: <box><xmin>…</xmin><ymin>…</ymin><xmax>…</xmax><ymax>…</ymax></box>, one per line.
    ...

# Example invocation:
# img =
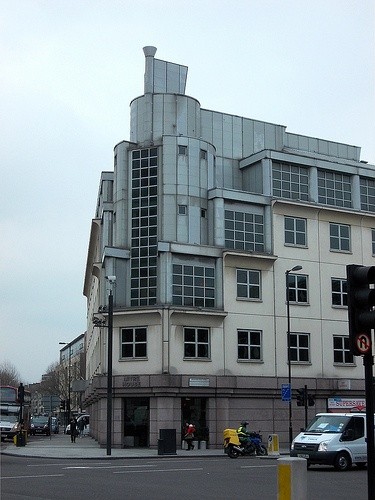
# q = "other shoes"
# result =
<box><xmin>243</xmin><ymin>446</ymin><xmax>249</xmax><ymax>453</ymax></box>
<box><xmin>74</xmin><ymin>441</ymin><xmax>76</xmax><ymax>443</ymax></box>
<box><xmin>186</xmin><ymin>449</ymin><xmax>190</xmax><ymax>451</ymax></box>
<box><xmin>192</xmin><ymin>445</ymin><xmax>194</xmax><ymax>450</ymax></box>
<box><xmin>71</xmin><ymin>442</ymin><xmax>73</xmax><ymax>443</ymax></box>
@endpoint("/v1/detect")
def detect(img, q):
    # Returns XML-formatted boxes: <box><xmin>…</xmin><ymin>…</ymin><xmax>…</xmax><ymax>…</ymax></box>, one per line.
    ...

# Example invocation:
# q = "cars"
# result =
<box><xmin>65</xmin><ymin>415</ymin><xmax>92</xmax><ymax>435</ymax></box>
<box><xmin>50</xmin><ymin>416</ymin><xmax>59</xmax><ymax>434</ymax></box>
<box><xmin>289</xmin><ymin>413</ymin><xmax>368</xmax><ymax>470</ymax></box>
<box><xmin>29</xmin><ymin>415</ymin><xmax>50</xmax><ymax>436</ymax></box>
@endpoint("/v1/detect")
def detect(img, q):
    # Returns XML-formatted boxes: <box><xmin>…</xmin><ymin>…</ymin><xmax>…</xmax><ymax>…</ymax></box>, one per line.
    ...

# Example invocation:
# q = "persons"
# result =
<box><xmin>54</xmin><ymin>417</ymin><xmax>62</xmax><ymax>434</ymax></box>
<box><xmin>183</xmin><ymin>419</ymin><xmax>195</xmax><ymax>451</ymax></box>
<box><xmin>237</xmin><ymin>421</ymin><xmax>250</xmax><ymax>453</ymax></box>
<box><xmin>71</xmin><ymin>419</ymin><xmax>76</xmax><ymax>443</ymax></box>
<box><xmin>78</xmin><ymin>418</ymin><xmax>85</xmax><ymax>436</ymax></box>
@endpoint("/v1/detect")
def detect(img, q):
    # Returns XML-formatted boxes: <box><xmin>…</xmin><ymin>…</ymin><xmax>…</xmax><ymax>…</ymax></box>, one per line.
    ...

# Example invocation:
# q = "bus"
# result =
<box><xmin>1</xmin><ymin>386</ymin><xmax>32</xmax><ymax>440</ymax></box>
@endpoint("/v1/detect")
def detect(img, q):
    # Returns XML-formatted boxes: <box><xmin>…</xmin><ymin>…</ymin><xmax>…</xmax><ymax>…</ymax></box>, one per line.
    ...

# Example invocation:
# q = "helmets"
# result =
<box><xmin>241</xmin><ymin>421</ymin><xmax>249</xmax><ymax>428</ymax></box>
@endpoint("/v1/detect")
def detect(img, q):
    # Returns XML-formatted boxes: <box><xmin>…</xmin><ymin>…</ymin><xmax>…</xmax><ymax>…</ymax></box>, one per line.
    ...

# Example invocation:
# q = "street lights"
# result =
<box><xmin>285</xmin><ymin>265</ymin><xmax>302</xmax><ymax>450</ymax></box>
<box><xmin>58</xmin><ymin>343</ymin><xmax>70</xmax><ymax>432</ymax></box>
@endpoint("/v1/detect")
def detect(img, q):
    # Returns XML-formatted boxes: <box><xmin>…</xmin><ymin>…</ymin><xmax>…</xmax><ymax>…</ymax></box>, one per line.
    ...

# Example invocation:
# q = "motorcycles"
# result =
<box><xmin>224</xmin><ymin>430</ymin><xmax>267</xmax><ymax>460</ymax></box>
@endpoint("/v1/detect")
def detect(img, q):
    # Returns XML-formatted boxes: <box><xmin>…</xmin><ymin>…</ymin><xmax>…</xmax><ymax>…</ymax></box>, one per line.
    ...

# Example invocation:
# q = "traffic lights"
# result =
<box><xmin>347</xmin><ymin>264</ymin><xmax>375</xmax><ymax>356</ymax></box>
<box><xmin>297</xmin><ymin>388</ymin><xmax>305</xmax><ymax>407</ymax></box>
<box><xmin>309</xmin><ymin>395</ymin><xmax>315</xmax><ymax>407</ymax></box>
<box><xmin>18</xmin><ymin>385</ymin><xmax>24</xmax><ymax>402</ymax></box>
<box><xmin>60</xmin><ymin>400</ymin><xmax>65</xmax><ymax>409</ymax></box>
